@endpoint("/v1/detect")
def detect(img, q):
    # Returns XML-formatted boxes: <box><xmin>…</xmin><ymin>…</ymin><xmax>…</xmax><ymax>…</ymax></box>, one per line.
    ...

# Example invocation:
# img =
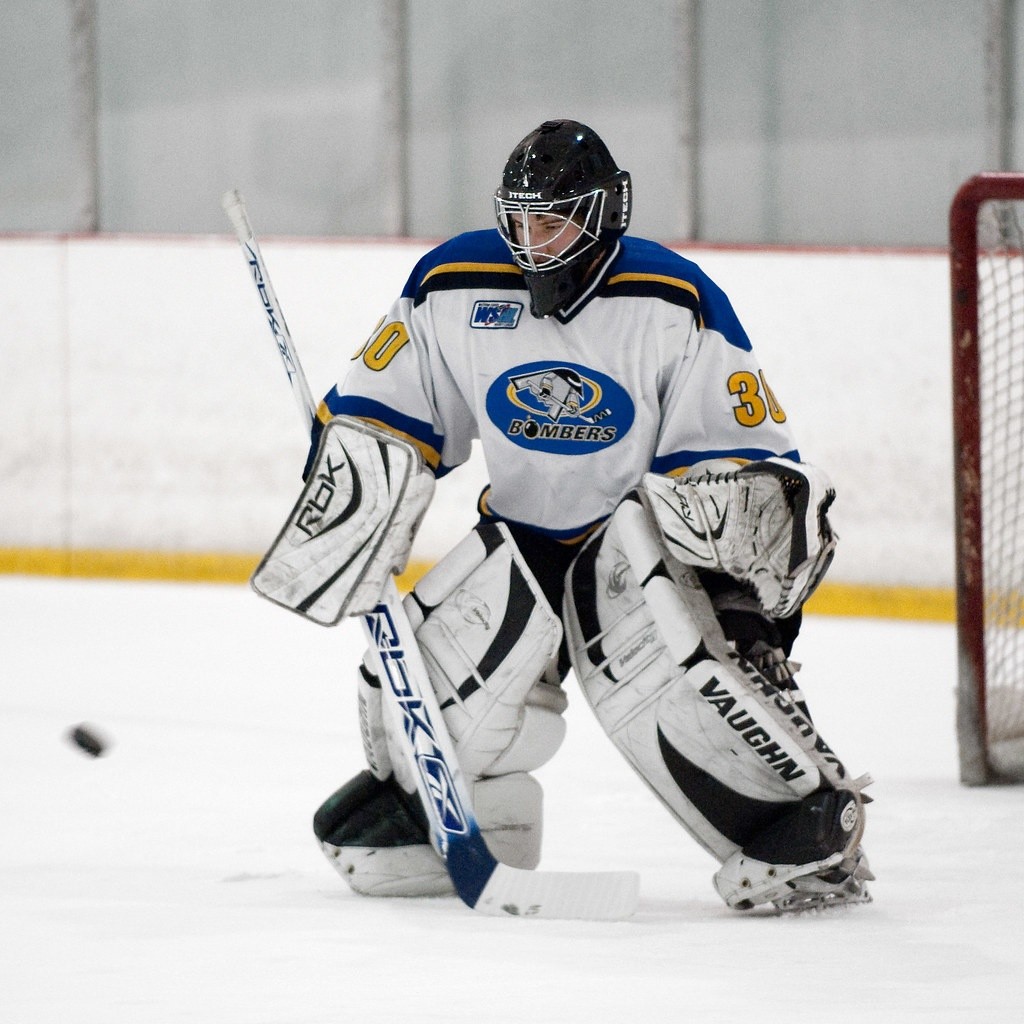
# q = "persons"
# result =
<box><xmin>253</xmin><ymin>118</ymin><xmax>875</xmax><ymax>915</ymax></box>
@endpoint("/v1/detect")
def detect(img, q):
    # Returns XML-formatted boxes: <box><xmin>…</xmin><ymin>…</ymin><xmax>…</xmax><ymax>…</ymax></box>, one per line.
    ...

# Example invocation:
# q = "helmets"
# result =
<box><xmin>492</xmin><ymin>120</ymin><xmax>632</xmax><ymax>320</ymax></box>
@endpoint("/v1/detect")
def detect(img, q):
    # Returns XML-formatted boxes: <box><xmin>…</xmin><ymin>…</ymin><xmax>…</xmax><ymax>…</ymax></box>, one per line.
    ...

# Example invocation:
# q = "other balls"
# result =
<box><xmin>71</xmin><ymin>726</ymin><xmax>103</xmax><ymax>757</ymax></box>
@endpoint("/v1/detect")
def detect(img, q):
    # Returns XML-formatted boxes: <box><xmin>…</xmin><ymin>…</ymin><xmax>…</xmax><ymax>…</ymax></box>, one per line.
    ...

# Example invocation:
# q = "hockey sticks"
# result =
<box><xmin>219</xmin><ymin>182</ymin><xmax>655</xmax><ymax>923</ymax></box>
